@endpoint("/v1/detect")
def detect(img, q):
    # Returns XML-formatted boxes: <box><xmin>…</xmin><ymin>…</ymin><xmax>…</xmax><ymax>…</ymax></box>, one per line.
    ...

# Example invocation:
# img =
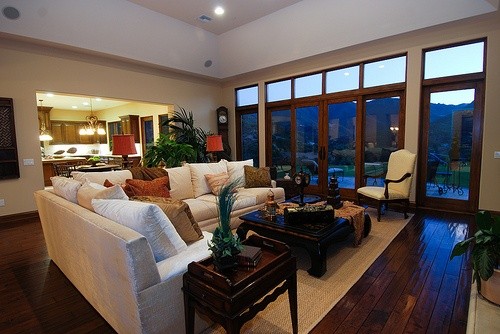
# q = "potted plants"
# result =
<box><xmin>448</xmin><ymin>210</ymin><xmax>500</xmax><ymax>306</ymax></box>
<box><xmin>205</xmin><ymin>171</ymin><xmax>244</xmax><ymax>273</ymax></box>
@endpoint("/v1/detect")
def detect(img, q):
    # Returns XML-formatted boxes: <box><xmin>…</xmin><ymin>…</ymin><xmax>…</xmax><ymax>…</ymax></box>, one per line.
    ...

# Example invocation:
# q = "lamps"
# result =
<box><xmin>112</xmin><ymin>130</ymin><xmax>137</xmax><ymax>170</ymax></box>
<box><xmin>38</xmin><ymin>99</ymin><xmax>54</xmax><ymax>142</ymax></box>
<box><xmin>206</xmin><ymin>133</ymin><xmax>223</xmax><ymax>164</ymax></box>
<box><xmin>79</xmin><ymin>97</ymin><xmax>106</xmax><ymax>136</ymax></box>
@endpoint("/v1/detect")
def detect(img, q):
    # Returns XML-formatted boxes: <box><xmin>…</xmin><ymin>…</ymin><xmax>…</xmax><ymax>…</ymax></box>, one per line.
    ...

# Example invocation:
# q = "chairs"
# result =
<box><xmin>357</xmin><ymin>149</ymin><xmax>417</xmax><ymax>222</ymax></box>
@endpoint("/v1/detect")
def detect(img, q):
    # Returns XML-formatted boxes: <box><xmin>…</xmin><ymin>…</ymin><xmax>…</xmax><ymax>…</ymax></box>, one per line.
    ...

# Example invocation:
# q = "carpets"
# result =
<box><xmin>196</xmin><ymin>206</ymin><xmax>415</xmax><ymax>334</ymax></box>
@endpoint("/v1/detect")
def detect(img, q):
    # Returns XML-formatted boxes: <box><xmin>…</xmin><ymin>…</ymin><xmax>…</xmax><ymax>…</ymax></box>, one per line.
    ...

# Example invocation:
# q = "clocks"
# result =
<box><xmin>293</xmin><ymin>166</ymin><xmax>311</xmax><ymax>206</ymax></box>
<box><xmin>216</xmin><ymin>106</ymin><xmax>231</xmax><ymax>162</ymax></box>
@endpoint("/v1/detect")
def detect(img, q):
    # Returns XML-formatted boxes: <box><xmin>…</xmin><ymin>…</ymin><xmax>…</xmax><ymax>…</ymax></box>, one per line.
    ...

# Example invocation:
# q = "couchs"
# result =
<box><xmin>34</xmin><ymin>159</ymin><xmax>285</xmax><ymax>334</ymax></box>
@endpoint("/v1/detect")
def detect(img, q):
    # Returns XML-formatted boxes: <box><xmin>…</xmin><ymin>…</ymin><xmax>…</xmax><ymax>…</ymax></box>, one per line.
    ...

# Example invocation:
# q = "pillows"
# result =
<box><xmin>204</xmin><ymin>172</ymin><xmax>239</xmax><ymax>195</ymax></box>
<box><xmin>243</xmin><ymin>165</ymin><xmax>273</xmax><ymax>188</ymax></box>
<box><xmin>128</xmin><ymin>196</ymin><xmax>204</xmax><ymax>242</ymax></box>
<box><xmin>104</xmin><ymin>179</ymin><xmax>135</xmax><ymax>200</ymax></box>
<box><xmin>124</xmin><ymin>176</ymin><xmax>172</xmax><ymax>198</ymax></box>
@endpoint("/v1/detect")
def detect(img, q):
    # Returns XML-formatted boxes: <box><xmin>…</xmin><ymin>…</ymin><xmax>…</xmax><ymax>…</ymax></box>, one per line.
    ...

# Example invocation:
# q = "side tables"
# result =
<box><xmin>181</xmin><ymin>234</ymin><xmax>299</xmax><ymax>334</ymax></box>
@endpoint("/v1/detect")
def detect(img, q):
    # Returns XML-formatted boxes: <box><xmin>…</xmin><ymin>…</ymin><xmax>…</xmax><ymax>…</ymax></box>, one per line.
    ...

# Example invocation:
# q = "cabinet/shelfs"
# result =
<box><xmin>119</xmin><ymin>114</ymin><xmax>140</xmax><ymax>144</ymax></box>
<box><xmin>37</xmin><ymin>106</ymin><xmax>107</xmax><ymax>145</ymax></box>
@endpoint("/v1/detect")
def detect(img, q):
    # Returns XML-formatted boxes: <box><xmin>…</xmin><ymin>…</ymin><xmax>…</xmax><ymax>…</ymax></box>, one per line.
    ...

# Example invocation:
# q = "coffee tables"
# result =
<box><xmin>235</xmin><ymin>193</ymin><xmax>372</xmax><ymax>278</ymax></box>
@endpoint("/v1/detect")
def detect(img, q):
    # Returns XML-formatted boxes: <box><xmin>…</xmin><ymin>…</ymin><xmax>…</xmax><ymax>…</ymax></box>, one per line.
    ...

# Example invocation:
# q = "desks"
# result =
<box><xmin>68</xmin><ymin>165</ymin><xmax>120</xmax><ymax>172</ymax></box>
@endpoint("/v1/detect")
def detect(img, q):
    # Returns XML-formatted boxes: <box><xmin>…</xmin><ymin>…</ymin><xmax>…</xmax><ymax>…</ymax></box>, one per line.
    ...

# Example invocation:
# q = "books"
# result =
<box><xmin>236</xmin><ymin>245</ymin><xmax>262</xmax><ymax>262</ymax></box>
<box><xmin>235</xmin><ymin>254</ymin><xmax>262</xmax><ymax>266</ymax></box>
